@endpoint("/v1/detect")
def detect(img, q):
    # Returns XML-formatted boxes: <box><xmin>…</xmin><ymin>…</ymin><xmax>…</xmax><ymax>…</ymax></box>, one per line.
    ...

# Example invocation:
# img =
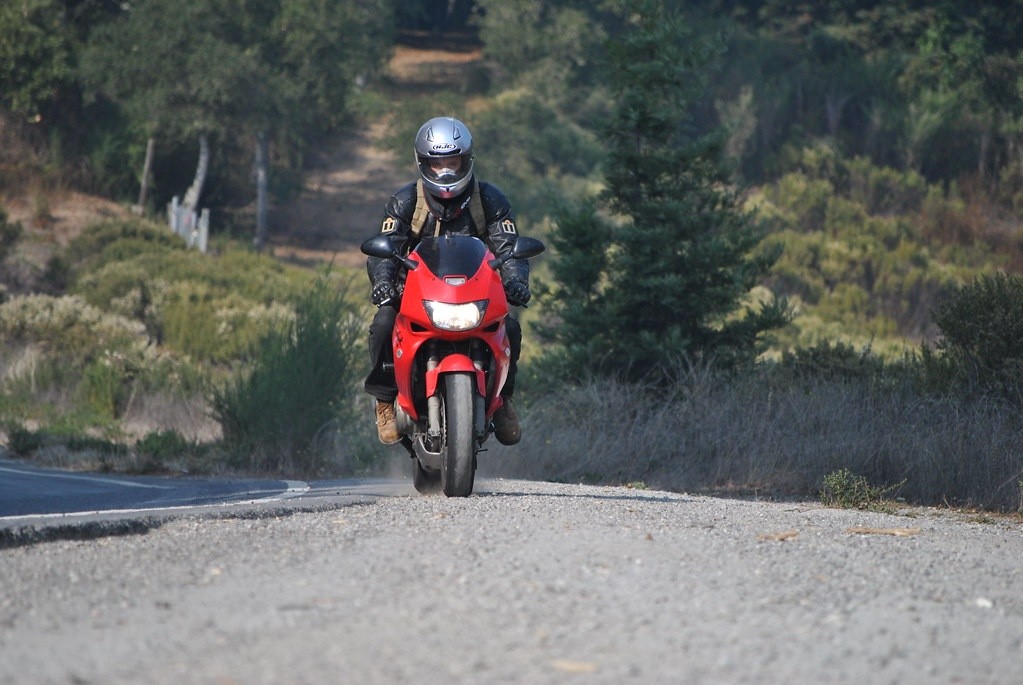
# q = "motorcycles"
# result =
<box><xmin>359</xmin><ymin>236</ymin><xmax>544</xmax><ymax>496</ymax></box>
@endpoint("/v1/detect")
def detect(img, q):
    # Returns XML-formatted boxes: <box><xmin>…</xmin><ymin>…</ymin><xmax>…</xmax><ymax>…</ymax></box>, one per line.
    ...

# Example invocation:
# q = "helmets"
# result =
<box><xmin>413</xmin><ymin>116</ymin><xmax>474</xmax><ymax>199</ymax></box>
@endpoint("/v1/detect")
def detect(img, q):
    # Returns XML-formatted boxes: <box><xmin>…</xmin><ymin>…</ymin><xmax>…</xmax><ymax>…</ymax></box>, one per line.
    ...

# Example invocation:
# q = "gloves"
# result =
<box><xmin>371</xmin><ymin>280</ymin><xmax>397</xmax><ymax>306</ymax></box>
<box><xmin>503</xmin><ymin>278</ymin><xmax>531</xmax><ymax>307</ymax></box>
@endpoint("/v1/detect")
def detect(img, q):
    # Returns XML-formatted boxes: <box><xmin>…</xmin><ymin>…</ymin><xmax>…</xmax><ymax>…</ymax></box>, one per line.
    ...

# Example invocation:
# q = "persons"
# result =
<box><xmin>364</xmin><ymin>117</ymin><xmax>531</xmax><ymax>446</ymax></box>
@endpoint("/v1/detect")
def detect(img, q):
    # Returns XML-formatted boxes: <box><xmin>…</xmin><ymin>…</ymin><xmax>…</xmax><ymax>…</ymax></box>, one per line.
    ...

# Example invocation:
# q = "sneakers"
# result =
<box><xmin>375</xmin><ymin>399</ymin><xmax>401</xmax><ymax>447</ymax></box>
<box><xmin>492</xmin><ymin>399</ymin><xmax>522</xmax><ymax>446</ymax></box>
